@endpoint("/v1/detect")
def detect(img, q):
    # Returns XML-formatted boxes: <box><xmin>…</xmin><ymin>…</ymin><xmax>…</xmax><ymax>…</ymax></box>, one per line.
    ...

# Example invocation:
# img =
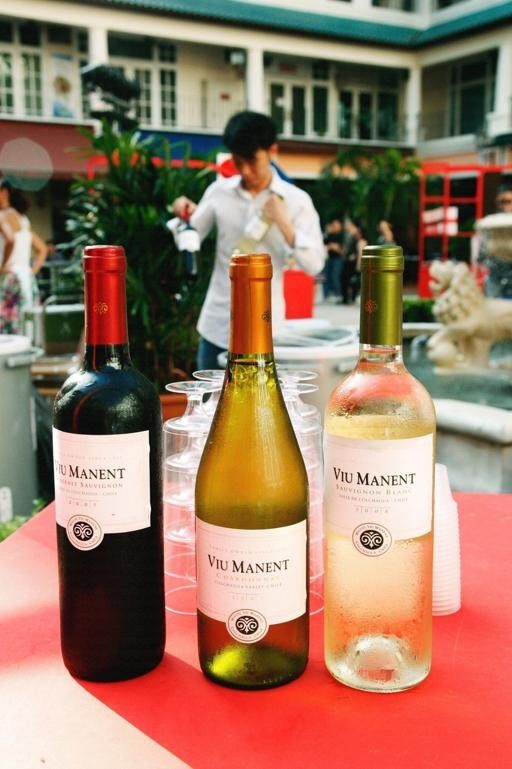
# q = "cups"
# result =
<box><xmin>431</xmin><ymin>463</ymin><xmax>462</xmax><ymax>616</ymax></box>
<box><xmin>164</xmin><ymin>368</ymin><xmax>323</xmax><ymax>605</ymax></box>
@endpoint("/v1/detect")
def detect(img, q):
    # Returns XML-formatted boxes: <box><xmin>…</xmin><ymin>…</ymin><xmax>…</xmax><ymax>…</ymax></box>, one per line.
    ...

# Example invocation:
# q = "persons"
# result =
<box><xmin>0</xmin><ymin>180</ymin><xmax>50</xmax><ymax>350</ymax></box>
<box><xmin>327</xmin><ymin>220</ymin><xmax>368</xmax><ymax>305</ymax></box>
<box><xmin>377</xmin><ymin>221</ymin><xmax>396</xmax><ymax>247</ymax></box>
<box><xmin>170</xmin><ymin>111</ymin><xmax>326</xmax><ymax>376</ymax></box>
<box><xmin>320</xmin><ymin>220</ymin><xmax>344</xmax><ymax>295</ymax></box>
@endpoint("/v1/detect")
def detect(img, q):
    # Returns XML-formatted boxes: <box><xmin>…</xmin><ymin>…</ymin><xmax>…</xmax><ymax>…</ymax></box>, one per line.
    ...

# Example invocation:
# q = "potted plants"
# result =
<box><xmin>35</xmin><ymin>129</ymin><xmax>219</xmax><ymax>423</ymax></box>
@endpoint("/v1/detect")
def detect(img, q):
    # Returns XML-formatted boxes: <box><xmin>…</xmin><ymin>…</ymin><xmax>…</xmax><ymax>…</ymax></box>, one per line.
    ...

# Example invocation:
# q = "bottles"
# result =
<box><xmin>51</xmin><ymin>248</ymin><xmax>165</xmax><ymax>684</ymax></box>
<box><xmin>324</xmin><ymin>245</ymin><xmax>436</xmax><ymax>694</ymax></box>
<box><xmin>196</xmin><ymin>255</ymin><xmax>310</xmax><ymax>689</ymax></box>
